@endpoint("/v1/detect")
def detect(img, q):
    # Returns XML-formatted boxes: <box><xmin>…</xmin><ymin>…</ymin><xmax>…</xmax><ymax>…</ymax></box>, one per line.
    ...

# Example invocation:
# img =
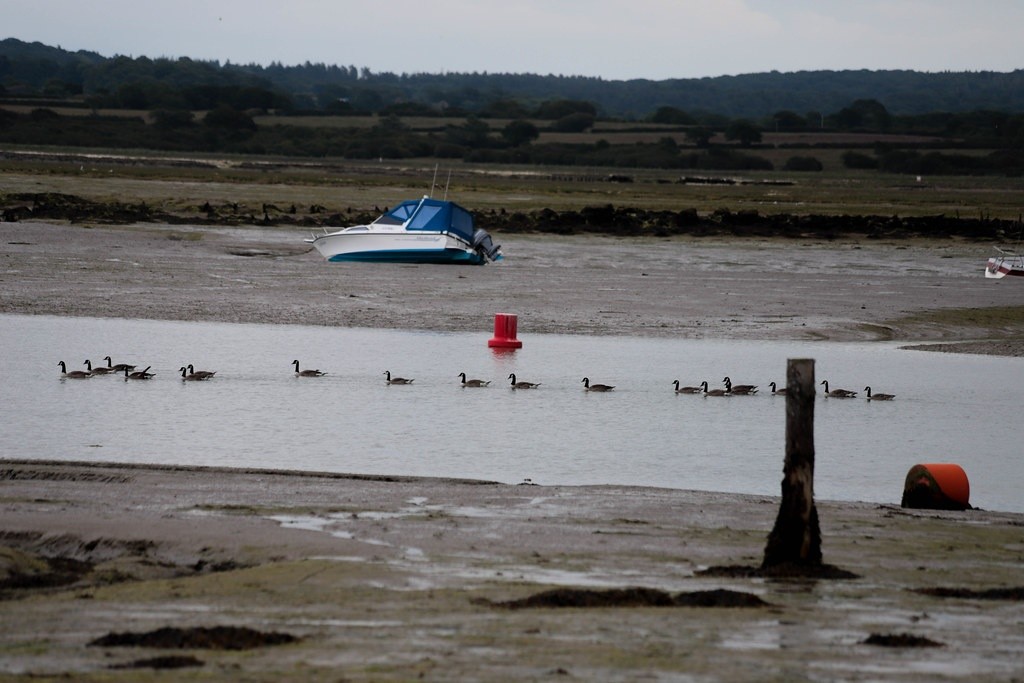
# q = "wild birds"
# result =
<box><xmin>291</xmin><ymin>360</ymin><xmax>328</xmax><ymax>377</ymax></box>
<box><xmin>863</xmin><ymin>386</ymin><xmax>896</xmax><ymax>401</ymax></box>
<box><xmin>672</xmin><ymin>379</ymin><xmax>704</xmax><ymax>394</ymax></box>
<box><xmin>56</xmin><ymin>356</ymin><xmax>156</xmax><ymax>380</ymax></box>
<box><xmin>508</xmin><ymin>373</ymin><xmax>542</xmax><ymax>390</ymax></box>
<box><xmin>178</xmin><ymin>364</ymin><xmax>217</xmax><ymax>381</ymax></box>
<box><xmin>768</xmin><ymin>382</ymin><xmax>787</xmax><ymax>396</ymax></box>
<box><xmin>820</xmin><ymin>380</ymin><xmax>858</xmax><ymax>398</ymax></box>
<box><xmin>382</xmin><ymin>371</ymin><xmax>415</xmax><ymax>386</ymax></box>
<box><xmin>458</xmin><ymin>372</ymin><xmax>491</xmax><ymax>388</ymax></box>
<box><xmin>700</xmin><ymin>376</ymin><xmax>760</xmax><ymax>397</ymax></box>
<box><xmin>581</xmin><ymin>377</ymin><xmax>616</xmax><ymax>392</ymax></box>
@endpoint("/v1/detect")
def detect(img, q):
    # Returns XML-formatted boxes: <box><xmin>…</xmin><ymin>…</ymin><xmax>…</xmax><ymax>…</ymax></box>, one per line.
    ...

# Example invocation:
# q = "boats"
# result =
<box><xmin>301</xmin><ymin>161</ymin><xmax>502</xmax><ymax>265</ymax></box>
<box><xmin>984</xmin><ymin>240</ymin><xmax>1024</xmax><ymax>281</ymax></box>
